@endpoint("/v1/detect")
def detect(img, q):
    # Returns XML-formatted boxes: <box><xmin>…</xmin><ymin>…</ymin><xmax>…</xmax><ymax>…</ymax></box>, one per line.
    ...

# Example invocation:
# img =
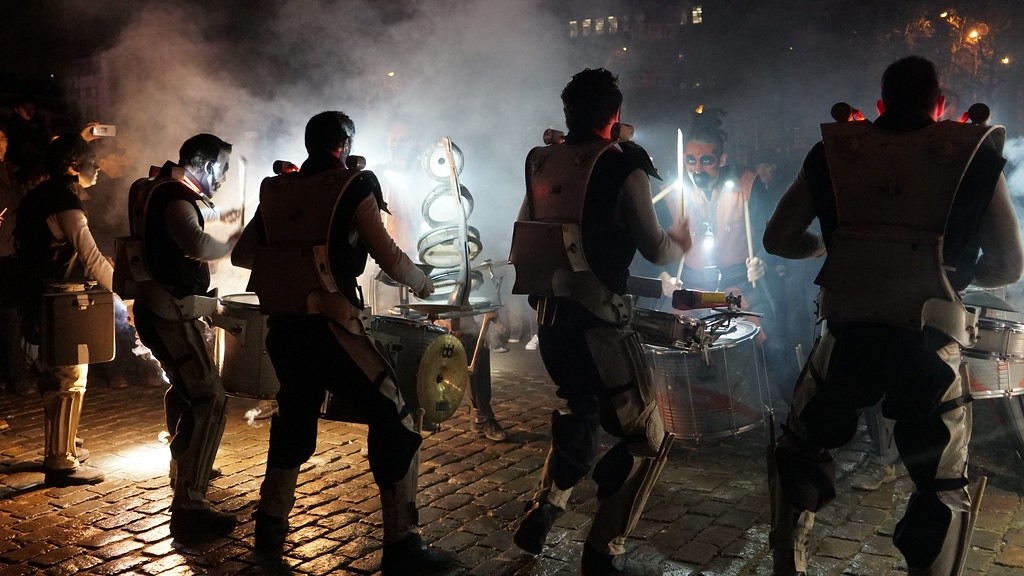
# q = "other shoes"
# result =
<box><xmin>143</xmin><ymin>375</ymin><xmax>163</xmax><ymax>386</ymax></box>
<box><xmin>380</xmin><ymin>533</ymin><xmax>457</xmax><ymax>575</ymax></box>
<box><xmin>495</xmin><ymin>347</ymin><xmax>509</xmax><ymax>352</ymax></box>
<box><xmin>252</xmin><ymin>511</ymin><xmax>288</xmax><ymax>551</ymax></box>
<box><xmin>44</xmin><ymin>469</ymin><xmax>103</xmax><ymax>486</ymax></box>
<box><xmin>170</xmin><ymin>467</ymin><xmax>221</xmax><ymax>487</ymax></box>
<box><xmin>579</xmin><ymin>544</ymin><xmax>660</xmax><ymax>576</ymax></box>
<box><xmin>13</xmin><ymin>382</ymin><xmax>35</xmax><ymax>396</ymax></box>
<box><xmin>525</xmin><ymin>336</ymin><xmax>539</xmax><ymax>350</ymax></box>
<box><xmin>171</xmin><ymin>508</ymin><xmax>236</xmax><ymax>537</ymax></box>
<box><xmin>512</xmin><ymin>506</ymin><xmax>548</xmax><ymax>553</ymax></box>
<box><xmin>76</xmin><ymin>446</ymin><xmax>90</xmax><ymax>462</ymax></box>
<box><xmin>109</xmin><ymin>375</ymin><xmax>127</xmax><ymax>390</ymax></box>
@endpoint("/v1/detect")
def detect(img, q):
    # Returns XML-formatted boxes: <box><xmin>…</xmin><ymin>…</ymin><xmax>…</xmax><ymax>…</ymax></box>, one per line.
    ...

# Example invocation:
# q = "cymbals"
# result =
<box><xmin>474</xmin><ymin>259</ymin><xmax>509</xmax><ymax>271</ymax></box>
<box><xmin>417</xmin><ymin>333</ymin><xmax>470</xmax><ymax>425</ymax></box>
<box><xmin>963</xmin><ymin>293</ymin><xmax>1019</xmax><ymax>313</ymax></box>
<box><xmin>394</xmin><ymin>303</ymin><xmax>475</xmax><ymax>315</ymax></box>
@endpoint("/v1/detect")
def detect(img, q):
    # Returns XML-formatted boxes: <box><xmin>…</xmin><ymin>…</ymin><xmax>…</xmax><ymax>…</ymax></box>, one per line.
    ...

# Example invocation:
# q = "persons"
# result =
<box><xmin>381</xmin><ymin>115</ymin><xmax>509</xmax><ymax>441</ymax></box>
<box><xmin>230</xmin><ymin>111</ymin><xmax>459</xmax><ymax>576</ymax></box>
<box><xmin>131</xmin><ymin>133</ymin><xmax>244</xmax><ymax>536</ymax></box>
<box><xmin>512</xmin><ymin>69</ymin><xmax>692</xmax><ymax>576</ymax></box>
<box><xmin>635</xmin><ymin>108</ymin><xmax>801</xmax><ymax>399</ymax></box>
<box><xmin>763</xmin><ymin>56</ymin><xmax>1024</xmax><ymax>576</ymax></box>
<box><xmin>84</xmin><ymin>139</ymin><xmax>161</xmax><ymax>388</ymax></box>
<box><xmin>0</xmin><ymin>122</ymin><xmax>16</xmax><ymax>432</ymax></box>
<box><xmin>15</xmin><ymin>135</ymin><xmax>114</xmax><ymax>484</ymax></box>
<box><xmin>753</xmin><ymin>149</ymin><xmax>785</xmax><ymax>198</ymax></box>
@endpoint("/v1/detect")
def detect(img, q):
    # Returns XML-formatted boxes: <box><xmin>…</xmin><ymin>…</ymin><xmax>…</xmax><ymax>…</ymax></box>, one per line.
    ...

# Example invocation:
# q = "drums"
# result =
<box><xmin>961</xmin><ymin>317</ymin><xmax>1024</xmax><ymax>364</ymax></box>
<box><xmin>645</xmin><ymin>321</ymin><xmax>773</xmax><ymax>442</ymax></box>
<box><xmin>960</xmin><ymin>355</ymin><xmax>1024</xmax><ymax>400</ymax></box>
<box><xmin>215</xmin><ymin>291</ymin><xmax>282</xmax><ymax>402</ymax></box>
<box><xmin>369</xmin><ymin>313</ymin><xmax>449</xmax><ymax>432</ymax></box>
<box><xmin>410</xmin><ymin>135</ymin><xmax>493</xmax><ymax>309</ymax></box>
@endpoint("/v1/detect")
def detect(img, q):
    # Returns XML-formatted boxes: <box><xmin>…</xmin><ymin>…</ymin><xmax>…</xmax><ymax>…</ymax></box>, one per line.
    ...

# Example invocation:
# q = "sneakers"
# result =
<box><xmin>472</xmin><ymin>420</ymin><xmax>509</xmax><ymax>440</ymax></box>
<box><xmin>243</xmin><ymin>400</ymin><xmax>279</xmax><ymax>420</ymax></box>
<box><xmin>848</xmin><ymin>459</ymin><xmax>897</xmax><ymax>490</ymax></box>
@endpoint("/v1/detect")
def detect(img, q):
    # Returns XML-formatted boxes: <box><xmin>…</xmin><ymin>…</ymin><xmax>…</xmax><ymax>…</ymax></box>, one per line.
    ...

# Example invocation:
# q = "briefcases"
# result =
<box><xmin>40</xmin><ymin>283</ymin><xmax>116</xmax><ymax>365</ymax></box>
